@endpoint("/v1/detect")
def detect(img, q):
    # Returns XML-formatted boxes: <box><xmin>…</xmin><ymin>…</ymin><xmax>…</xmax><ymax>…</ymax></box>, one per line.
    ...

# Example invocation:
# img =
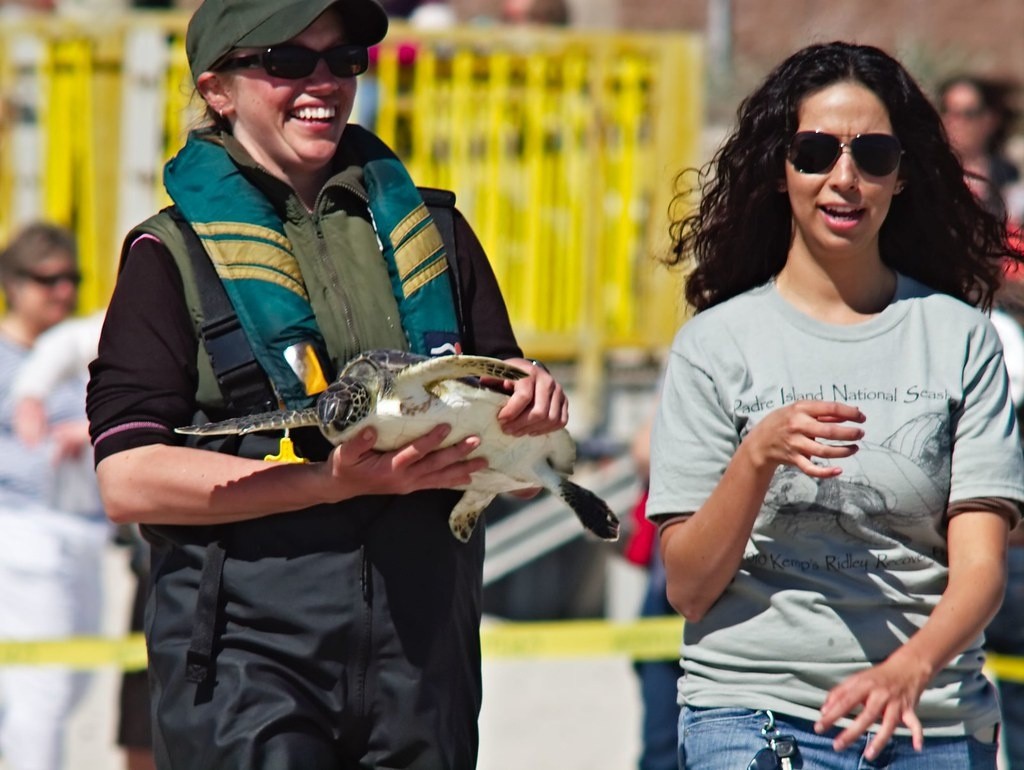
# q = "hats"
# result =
<box><xmin>185</xmin><ymin>0</ymin><xmax>389</xmax><ymax>99</ymax></box>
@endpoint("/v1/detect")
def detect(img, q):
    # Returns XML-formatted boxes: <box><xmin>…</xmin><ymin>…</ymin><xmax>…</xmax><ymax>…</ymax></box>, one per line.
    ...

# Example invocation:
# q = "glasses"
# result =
<box><xmin>214</xmin><ymin>43</ymin><xmax>369</xmax><ymax>80</ymax></box>
<box><xmin>940</xmin><ymin>106</ymin><xmax>987</xmax><ymax>121</ymax></box>
<box><xmin>11</xmin><ymin>267</ymin><xmax>81</xmax><ymax>288</ymax></box>
<box><xmin>776</xmin><ymin>130</ymin><xmax>907</xmax><ymax>178</ymax></box>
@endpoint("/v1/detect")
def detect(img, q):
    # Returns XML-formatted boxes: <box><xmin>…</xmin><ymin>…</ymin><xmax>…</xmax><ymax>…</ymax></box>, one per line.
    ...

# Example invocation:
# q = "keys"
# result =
<box><xmin>747</xmin><ymin>748</ymin><xmax>778</xmax><ymax>770</ymax></box>
<box><xmin>775</xmin><ymin>739</ymin><xmax>795</xmax><ymax>770</ymax></box>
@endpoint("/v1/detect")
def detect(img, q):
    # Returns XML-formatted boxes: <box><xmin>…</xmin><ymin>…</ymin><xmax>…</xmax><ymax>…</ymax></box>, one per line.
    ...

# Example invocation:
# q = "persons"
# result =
<box><xmin>1</xmin><ymin>223</ymin><xmax>154</xmax><ymax>770</ymax></box>
<box><xmin>645</xmin><ymin>42</ymin><xmax>1024</xmax><ymax>770</ymax></box>
<box><xmin>628</xmin><ymin>389</ymin><xmax>690</xmax><ymax>769</ymax></box>
<box><xmin>86</xmin><ymin>0</ymin><xmax>569</xmax><ymax>770</ymax></box>
<box><xmin>933</xmin><ymin>69</ymin><xmax>1024</xmax><ymax>402</ymax></box>
